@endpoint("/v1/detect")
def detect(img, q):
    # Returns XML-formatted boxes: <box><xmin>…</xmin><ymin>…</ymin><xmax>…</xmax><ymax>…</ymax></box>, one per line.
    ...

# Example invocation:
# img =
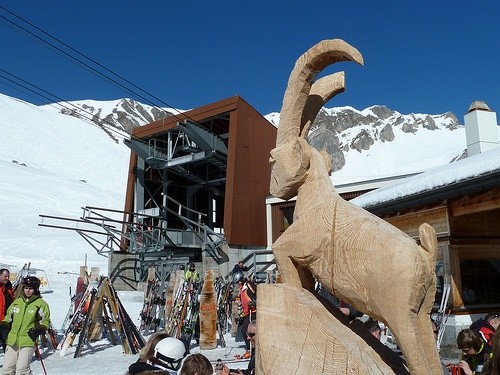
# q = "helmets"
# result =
<box><xmin>232</xmin><ymin>272</ymin><xmax>243</xmax><ymax>283</ymax></box>
<box><xmin>155</xmin><ymin>337</ymin><xmax>186</xmax><ymax>369</ymax></box>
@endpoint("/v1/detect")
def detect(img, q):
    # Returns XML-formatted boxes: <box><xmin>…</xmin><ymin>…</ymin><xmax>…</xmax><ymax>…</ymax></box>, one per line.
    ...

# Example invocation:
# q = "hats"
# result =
<box><xmin>21</xmin><ymin>276</ymin><xmax>40</xmax><ymax>294</ymax></box>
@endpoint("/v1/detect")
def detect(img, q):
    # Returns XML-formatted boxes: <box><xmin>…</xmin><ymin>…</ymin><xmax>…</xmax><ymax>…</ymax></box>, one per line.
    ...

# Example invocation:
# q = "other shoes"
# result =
<box><xmin>243</xmin><ymin>352</ymin><xmax>250</xmax><ymax>358</ymax></box>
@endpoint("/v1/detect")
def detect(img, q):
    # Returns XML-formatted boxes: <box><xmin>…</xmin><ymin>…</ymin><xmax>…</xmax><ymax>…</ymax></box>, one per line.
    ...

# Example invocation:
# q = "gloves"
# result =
<box><xmin>26</xmin><ymin>329</ymin><xmax>39</xmax><ymax>340</ymax></box>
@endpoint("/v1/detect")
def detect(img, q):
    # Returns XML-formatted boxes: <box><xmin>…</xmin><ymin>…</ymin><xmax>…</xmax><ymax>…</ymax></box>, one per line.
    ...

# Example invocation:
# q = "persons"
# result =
<box><xmin>0</xmin><ymin>269</ymin><xmax>15</xmax><ymax>356</ymax></box>
<box><xmin>446</xmin><ymin>312</ymin><xmax>500</xmax><ymax>375</ymax></box>
<box><xmin>215</xmin><ymin>320</ymin><xmax>256</xmax><ymax>375</ymax></box>
<box><xmin>126</xmin><ymin>333</ymin><xmax>213</xmax><ymax>375</ymax></box>
<box><xmin>319</xmin><ymin>285</ymin><xmax>381</xmax><ymax>341</ymax></box>
<box><xmin>229</xmin><ymin>273</ymin><xmax>257</xmax><ymax>358</ymax></box>
<box><xmin>0</xmin><ymin>276</ymin><xmax>50</xmax><ymax>375</ymax></box>
<box><xmin>231</xmin><ymin>261</ymin><xmax>248</xmax><ymax>274</ymax></box>
<box><xmin>185</xmin><ymin>262</ymin><xmax>199</xmax><ymax>283</ymax></box>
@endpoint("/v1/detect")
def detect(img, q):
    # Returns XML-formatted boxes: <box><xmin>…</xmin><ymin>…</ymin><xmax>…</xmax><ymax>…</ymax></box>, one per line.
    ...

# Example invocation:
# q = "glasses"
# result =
<box><xmin>246</xmin><ymin>332</ymin><xmax>255</xmax><ymax>338</ymax></box>
<box><xmin>488</xmin><ymin>312</ymin><xmax>499</xmax><ymax>323</ymax></box>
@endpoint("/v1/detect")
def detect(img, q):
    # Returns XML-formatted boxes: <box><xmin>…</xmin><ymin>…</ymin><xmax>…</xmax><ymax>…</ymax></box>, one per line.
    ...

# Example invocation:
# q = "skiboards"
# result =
<box><xmin>57</xmin><ymin>277</ymin><xmax>230</xmax><ymax>359</ymax></box>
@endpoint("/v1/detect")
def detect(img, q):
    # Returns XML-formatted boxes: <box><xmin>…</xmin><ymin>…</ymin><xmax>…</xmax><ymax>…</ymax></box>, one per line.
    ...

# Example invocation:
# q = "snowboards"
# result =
<box><xmin>75</xmin><ymin>278</ymin><xmax>85</xmax><ymax>313</ymax></box>
